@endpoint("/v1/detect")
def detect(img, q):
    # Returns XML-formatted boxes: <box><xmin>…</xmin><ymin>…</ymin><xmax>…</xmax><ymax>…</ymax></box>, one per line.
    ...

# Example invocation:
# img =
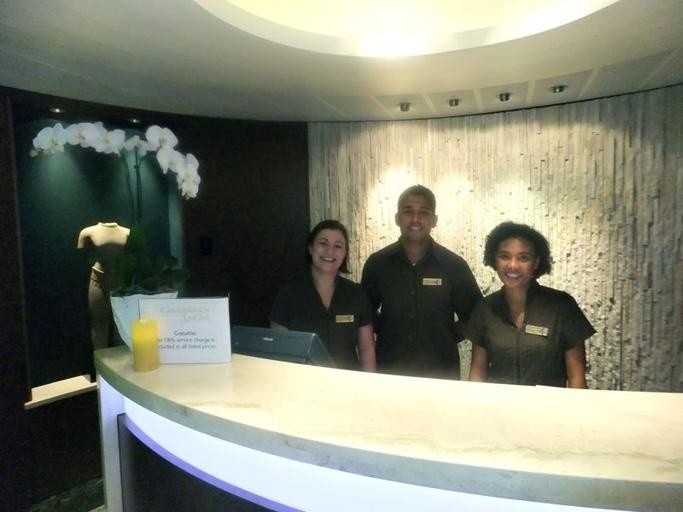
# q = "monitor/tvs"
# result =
<box><xmin>231</xmin><ymin>325</ymin><xmax>336</xmax><ymax>368</ymax></box>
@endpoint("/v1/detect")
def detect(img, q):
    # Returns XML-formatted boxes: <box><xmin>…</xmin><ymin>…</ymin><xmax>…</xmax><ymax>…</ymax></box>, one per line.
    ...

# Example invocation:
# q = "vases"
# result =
<box><xmin>110</xmin><ymin>291</ymin><xmax>179</xmax><ymax>355</ymax></box>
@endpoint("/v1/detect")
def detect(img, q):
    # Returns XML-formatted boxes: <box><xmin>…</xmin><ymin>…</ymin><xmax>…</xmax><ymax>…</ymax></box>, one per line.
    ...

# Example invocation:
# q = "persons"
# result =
<box><xmin>464</xmin><ymin>221</ymin><xmax>597</xmax><ymax>389</ymax></box>
<box><xmin>270</xmin><ymin>219</ymin><xmax>376</xmax><ymax>371</ymax></box>
<box><xmin>362</xmin><ymin>186</ymin><xmax>485</xmax><ymax>379</ymax></box>
<box><xmin>77</xmin><ymin>222</ymin><xmax>130</xmax><ymax>349</ymax></box>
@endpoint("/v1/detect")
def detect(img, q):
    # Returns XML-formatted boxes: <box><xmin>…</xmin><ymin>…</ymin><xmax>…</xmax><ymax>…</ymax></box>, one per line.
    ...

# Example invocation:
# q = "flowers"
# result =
<box><xmin>29</xmin><ymin>120</ymin><xmax>200</xmax><ymax>295</ymax></box>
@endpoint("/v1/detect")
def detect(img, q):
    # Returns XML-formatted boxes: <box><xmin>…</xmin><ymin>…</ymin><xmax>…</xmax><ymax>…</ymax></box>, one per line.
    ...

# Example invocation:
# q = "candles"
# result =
<box><xmin>132</xmin><ymin>318</ymin><xmax>159</xmax><ymax>372</ymax></box>
